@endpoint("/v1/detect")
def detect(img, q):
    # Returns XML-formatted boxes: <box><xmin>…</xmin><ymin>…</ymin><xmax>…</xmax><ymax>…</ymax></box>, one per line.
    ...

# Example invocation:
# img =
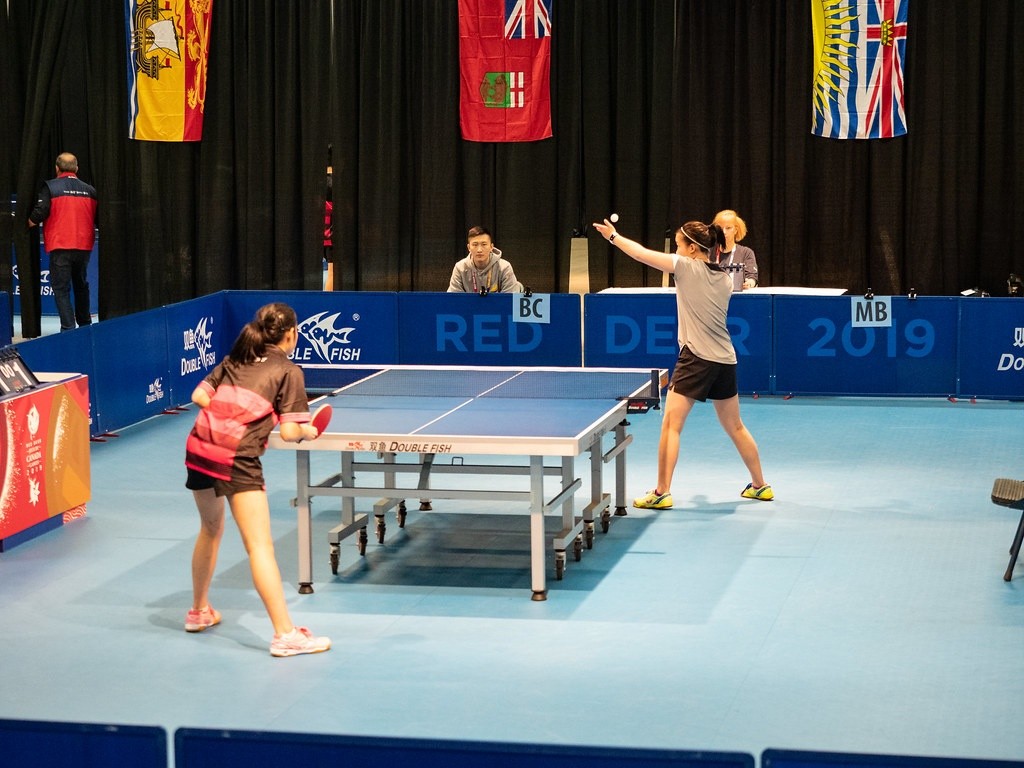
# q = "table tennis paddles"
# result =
<box><xmin>294</xmin><ymin>403</ymin><xmax>333</xmax><ymax>444</ymax></box>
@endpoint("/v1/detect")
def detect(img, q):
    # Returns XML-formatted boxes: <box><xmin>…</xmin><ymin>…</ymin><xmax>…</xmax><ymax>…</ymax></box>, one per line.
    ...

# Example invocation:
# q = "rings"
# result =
<box><xmin>747</xmin><ymin>284</ymin><xmax>748</xmax><ymax>286</ymax></box>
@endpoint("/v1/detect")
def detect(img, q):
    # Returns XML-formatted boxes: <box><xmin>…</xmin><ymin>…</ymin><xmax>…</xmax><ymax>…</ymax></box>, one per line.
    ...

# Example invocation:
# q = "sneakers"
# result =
<box><xmin>741</xmin><ymin>483</ymin><xmax>774</xmax><ymax>499</ymax></box>
<box><xmin>184</xmin><ymin>604</ymin><xmax>221</xmax><ymax>632</ymax></box>
<box><xmin>633</xmin><ymin>489</ymin><xmax>673</xmax><ymax>508</ymax></box>
<box><xmin>270</xmin><ymin>627</ymin><xmax>331</xmax><ymax>656</ymax></box>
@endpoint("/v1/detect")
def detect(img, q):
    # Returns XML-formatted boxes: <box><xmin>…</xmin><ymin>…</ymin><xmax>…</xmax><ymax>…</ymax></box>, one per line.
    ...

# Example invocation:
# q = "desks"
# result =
<box><xmin>0</xmin><ymin>372</ymin><xmax>91</xmax><ymax>552</ymax></box>
<box><xmin>598</xmin><ymin>286</ymin><xmax>849</xmax><ymax>296</ymax></box>
<box><xmin>265</xmin><ymin>365</ymin><xmax>670</xmax><ymax>602</ymax></box>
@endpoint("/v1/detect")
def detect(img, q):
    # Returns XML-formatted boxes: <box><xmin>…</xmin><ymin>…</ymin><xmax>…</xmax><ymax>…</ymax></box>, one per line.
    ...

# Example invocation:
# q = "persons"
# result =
<box><xmin>185</xmin><ymin>303</ymin><xmax>333</xmax><ymax>658</ymax></box>
<box><xmin>593</xmin><ymin>218</ymin><xmax>774</xmax><ymax>508</ymax></box>
<box><xmin>29</xmin><ymin>152</ymin><xmax>97</xmax><ymax>333</ymax></box>
<box><xmin>711</xmin><ymin>209</ymin><xmax>759</xmax><ymax>290</ymax></box>
<box><xmin>447</xmin><ymin>226</ymin><xmax>520</xmax><ymax>294</ymax></box>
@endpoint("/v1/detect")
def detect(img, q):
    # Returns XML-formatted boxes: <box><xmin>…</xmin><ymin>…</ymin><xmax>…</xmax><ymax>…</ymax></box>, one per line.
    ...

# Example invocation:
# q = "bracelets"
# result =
<box><xmin>750</xmin><ymin>283</ymin><xmax>752</xmax><ymax>287</ymax></box>
<box><xmin>609</xmin><ymin>230</ymin><xmax>618</xmax><ymax>243</ymax></box>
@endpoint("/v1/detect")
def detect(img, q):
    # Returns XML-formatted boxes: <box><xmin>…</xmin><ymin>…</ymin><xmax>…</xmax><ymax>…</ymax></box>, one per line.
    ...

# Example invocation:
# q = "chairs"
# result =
<box><xmin>992</xmin><ymin>479</ymin><xmax>1024</xmax><ymax>582</ymax></box>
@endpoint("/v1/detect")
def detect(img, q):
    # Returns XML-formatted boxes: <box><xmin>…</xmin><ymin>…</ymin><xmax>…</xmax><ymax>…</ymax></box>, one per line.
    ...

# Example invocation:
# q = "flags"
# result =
<box><xmin>124</xmin><ymin>0</ymin><xmax>213</xmax><ymax>142</ymax></box>
<box><xmin>458</xmin><ymin>0</ymin><xmax>553</xmax><ymax>141</ymax></box>
<box><xmin>810</xmin><ymin>0</ymin><xmax>909</xmax><ymax>139</ymax></box>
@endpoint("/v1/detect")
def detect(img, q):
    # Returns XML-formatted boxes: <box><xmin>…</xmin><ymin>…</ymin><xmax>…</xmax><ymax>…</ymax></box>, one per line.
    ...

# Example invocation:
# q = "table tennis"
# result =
<box><xmin>610</xmin><ymin>213</ymin><xmax>619</xmax><ymax>223</ymax></box>
